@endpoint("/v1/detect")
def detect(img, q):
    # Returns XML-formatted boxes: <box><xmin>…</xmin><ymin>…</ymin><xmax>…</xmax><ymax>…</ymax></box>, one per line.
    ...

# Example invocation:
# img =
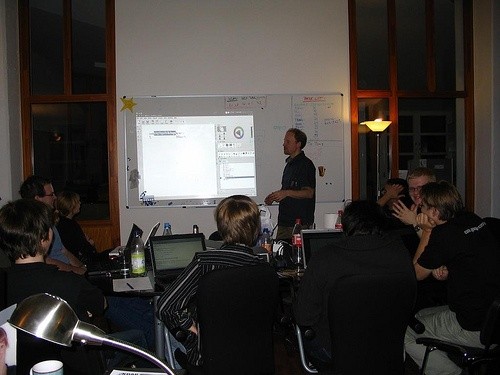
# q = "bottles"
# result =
<box><xmin>162</xmin><ymin>223</ymin><xmax>172</xmax><ymax>236</ymax></box>
<box><xmin>335</xmin><ymin>209</ymin><xmax>343</xmax><ymax>229</ymax></box>
<box><xmin>260</xmin><ymin>228</ymin><xmax>273</xmax><ymax>263</ymax></box>
<box><xmin>130</xmin><ymin>230</ymin><xmax>146</xmax><ymax>277</ymax></box>
<box><xmin>291</xmin><ymin>219</ymin><xmax>303</xmax><ymax>263</ymax></box>
<box><xmin>193</xmin><ymin>224</ymin><xmax>199</xmax><ymax>234</ymax></box>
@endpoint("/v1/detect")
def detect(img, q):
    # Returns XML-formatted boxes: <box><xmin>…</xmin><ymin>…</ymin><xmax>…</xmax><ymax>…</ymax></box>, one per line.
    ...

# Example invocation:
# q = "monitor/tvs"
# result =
<box><xmin>300</xmin><ymin>230</ymin><xmax>344</xmax><ymax>270</ymax></box>
<box><xmin>149</xmin><ymin>233</ymin><xmax>207</xmax><ymax>277</ymax></box>
<box><xmin>143</xmin><ymin>221</ymin><xmax>161</xmax><ymax>247</ymax></box>
<box><xmin>124</xmin><ymin>223</ymin><xmax>143</xmax><ymax>251</ymax></box>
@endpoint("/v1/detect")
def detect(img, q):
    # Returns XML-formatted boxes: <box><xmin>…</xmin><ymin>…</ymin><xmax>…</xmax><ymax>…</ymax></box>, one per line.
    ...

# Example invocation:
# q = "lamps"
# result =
<box><xmin>361</xmin><ymin>119</ymin><xmax>392</xmax><ymax>200</ymax></box>
<box><xmin>7</xmin><ymin>293</ymin><xmax>175</xmax><ymax>375</ymax></box>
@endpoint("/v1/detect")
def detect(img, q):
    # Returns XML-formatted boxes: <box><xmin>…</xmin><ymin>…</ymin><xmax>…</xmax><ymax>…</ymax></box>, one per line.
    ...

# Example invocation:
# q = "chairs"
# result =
<box><xmin>416</xmin><ymin>296</ymin><xmax>500</xmax><ymax>375</ymax></box>
<box><xmin>290</xmin><ymin>274</ymin><xmax>425</xmax><ymax>375</ymax></box>
<box><xmin>165</xmin><ymin>263</ymin><xmax>281</xmax><ymax>374</ymax></box>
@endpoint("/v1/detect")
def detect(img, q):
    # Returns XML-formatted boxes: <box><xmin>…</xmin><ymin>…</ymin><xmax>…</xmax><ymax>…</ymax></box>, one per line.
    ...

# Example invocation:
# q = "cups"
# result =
<box><xmin>29</xmin><ymin>360</ymin><xmax>64</xmax><ymax>375</ymax></box>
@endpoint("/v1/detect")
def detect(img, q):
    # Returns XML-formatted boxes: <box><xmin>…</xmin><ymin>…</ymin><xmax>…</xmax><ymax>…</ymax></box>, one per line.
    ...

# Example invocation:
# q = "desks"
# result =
<box><xmin>84</xmin><ymin>257</ymin><xmax>302</xmax><ymax>360</ymax></box>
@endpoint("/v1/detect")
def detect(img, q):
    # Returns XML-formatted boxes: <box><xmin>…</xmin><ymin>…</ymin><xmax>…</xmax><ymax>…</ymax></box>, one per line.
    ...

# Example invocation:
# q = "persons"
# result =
<box><xmin>154</xmin><ymin>194</ymin><xmax>276</xmax><ymax>375</ymax></box>
<box><xmin>0</xmin><ymin>198</ymin><xmax>155</xmax><ymax>375</ymax></box>
<box><xmin>265</xmin><ymin>128</ymin><xmax>315</xmax><ymax>244</ymax></box>
<box><xmin>380</xmin><ymin>168</ymin><xmax>500</xmax><ymax>375</ymax></box>
<box><xmin>53</xmin><ymin>187</ymin><xmax>124</xmax><ymax>266</ymax></box>
<box><xmin>293</xmin><ymin>200</ymin><xmax>418</xmax><ymax>375</ymax></box>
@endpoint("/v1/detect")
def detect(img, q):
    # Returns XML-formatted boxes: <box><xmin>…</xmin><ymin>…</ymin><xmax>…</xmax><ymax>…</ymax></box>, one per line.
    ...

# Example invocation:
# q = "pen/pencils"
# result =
<box><xmin>126</xmin><ymin>283</ymin><xmax>134</xmax><ymax>290</ymax></box>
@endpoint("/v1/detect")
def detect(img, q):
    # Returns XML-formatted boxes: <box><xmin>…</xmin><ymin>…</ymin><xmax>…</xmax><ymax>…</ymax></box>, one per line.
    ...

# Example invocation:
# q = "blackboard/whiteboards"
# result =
<box><xmin>121</xmin><ymin>94</ymin><xmax>345</xmax><ymax>210</ymax></box>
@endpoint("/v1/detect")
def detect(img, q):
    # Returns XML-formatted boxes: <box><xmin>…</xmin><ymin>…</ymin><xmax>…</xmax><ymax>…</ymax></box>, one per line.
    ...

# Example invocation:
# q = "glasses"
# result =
<box><xmin>409</xmin><ymin>185</ymin><xmax>422</xmax><ymax>193</ymax></box>
<box><xmin>44</xmin><ymin>191</ymin><xmax>55</xmax><ymax>196</ymax></box>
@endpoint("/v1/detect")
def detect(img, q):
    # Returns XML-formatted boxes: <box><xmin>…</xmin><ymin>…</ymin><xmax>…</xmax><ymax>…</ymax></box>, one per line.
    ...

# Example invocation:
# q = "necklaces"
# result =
<box><xmin>19</xmin><ymin>175</ymin><xmax>157</xmax><ymax>354</ymax></box>
